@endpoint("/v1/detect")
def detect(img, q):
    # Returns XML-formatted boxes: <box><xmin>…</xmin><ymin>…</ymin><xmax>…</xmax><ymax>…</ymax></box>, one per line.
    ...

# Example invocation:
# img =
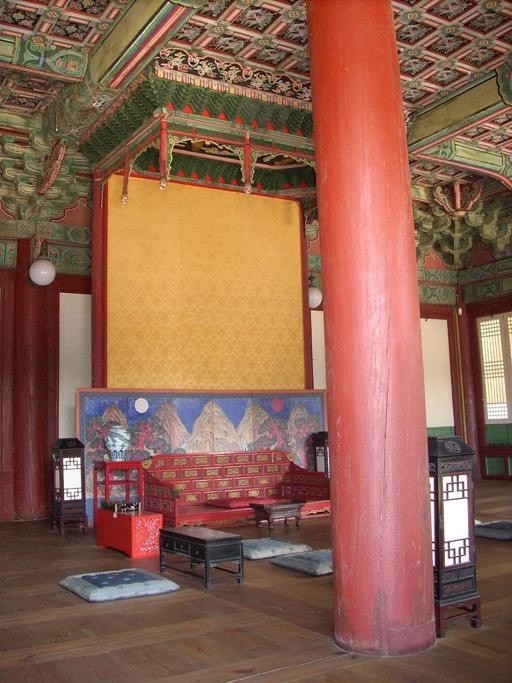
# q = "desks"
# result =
<box><xmin>158</xmin><ymin>525</ymin><xmax>245</xmax><ymax>590</ymax></box>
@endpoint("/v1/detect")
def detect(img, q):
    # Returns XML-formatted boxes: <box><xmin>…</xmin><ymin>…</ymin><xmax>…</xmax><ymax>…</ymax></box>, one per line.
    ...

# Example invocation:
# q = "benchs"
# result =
<box><xmin>140</xmin><ymin>448</ymin><xmax>335</xmax><ymax>530</ymax></box>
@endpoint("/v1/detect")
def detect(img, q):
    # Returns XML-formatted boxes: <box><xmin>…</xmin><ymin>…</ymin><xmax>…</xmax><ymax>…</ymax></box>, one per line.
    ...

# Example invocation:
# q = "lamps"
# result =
<box><xmin>30</xmin><ymin>233</ymin><xmax>57</xmax><ymax>285</ymax></box>
<box><xmin>308</xmin><ymin>264</ymin><xmax>324</xmax><ymax>309</ymax></box>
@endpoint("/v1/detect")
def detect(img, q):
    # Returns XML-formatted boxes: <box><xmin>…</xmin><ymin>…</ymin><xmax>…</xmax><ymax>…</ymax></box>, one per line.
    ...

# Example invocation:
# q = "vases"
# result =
<box><xmin>104</xmin><ymin>425</ymin><xmax>131</xmax><ymax>461</ymax></box>
